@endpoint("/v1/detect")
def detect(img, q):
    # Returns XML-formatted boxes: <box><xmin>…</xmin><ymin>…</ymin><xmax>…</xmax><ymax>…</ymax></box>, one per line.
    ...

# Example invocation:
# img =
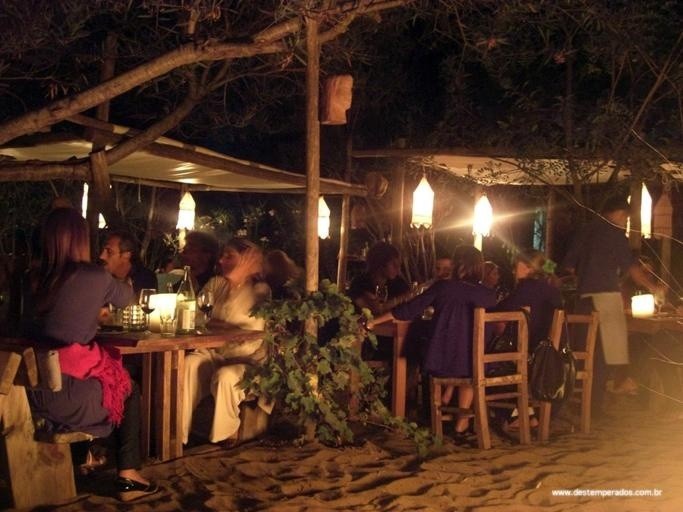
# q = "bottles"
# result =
<box><xmin>175</xmin><ymin>265</ymin><xmax>196</xmax><ymax>336</ymax></box>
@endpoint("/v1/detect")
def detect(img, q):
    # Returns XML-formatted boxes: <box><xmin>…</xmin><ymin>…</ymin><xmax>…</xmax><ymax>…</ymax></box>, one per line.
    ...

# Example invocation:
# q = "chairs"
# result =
<box><xmin>429</xmin><ymin>305</ymin><xmax>532</xmax><ymax>451</ymax></box>
<box><xmin>527</xmin><ymin>308</ymin><xmax>600</xmax><ymax>445</ymax></box>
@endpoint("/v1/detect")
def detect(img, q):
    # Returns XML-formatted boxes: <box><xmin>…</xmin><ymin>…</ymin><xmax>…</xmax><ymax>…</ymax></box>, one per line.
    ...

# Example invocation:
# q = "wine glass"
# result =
<box><xmin>654</xmin><ymin>289</ymin><xmax>665</xmax><ymax>314</ymax></box>
<box><xmin>197</xmin><ymin>289</ymin><xmax>214</xmax><ymax>334</ymax></box>
<box><xmin>138</xmin><ymin>288</ymin><xmax>156</xmax><ymax>336</ymax></box>
<box><xmin>635</xmin><ymin>290</ymin><xmax>641</xmax><ymax>296</ymax></box>
<box><xmin>375</xmin><ymin>285</ymin><xmax>388</xmax><ymax>314</ymax></box>
<box><xmin>421</xmin><ymin>287</ymin><xmax>432</xmax><ymax>320</ymax></box>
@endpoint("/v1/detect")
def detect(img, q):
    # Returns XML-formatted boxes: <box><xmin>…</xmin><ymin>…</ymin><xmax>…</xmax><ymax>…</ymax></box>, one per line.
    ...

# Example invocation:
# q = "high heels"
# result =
<box><xmin>75</xmin><ymin>453</ymin><xmax>106</xmax><ymax>476</ymax></box>
<box><xmin>115</xmin><ymin>476</ymin><xmax>159</xmax><ymax>501</ymax></box>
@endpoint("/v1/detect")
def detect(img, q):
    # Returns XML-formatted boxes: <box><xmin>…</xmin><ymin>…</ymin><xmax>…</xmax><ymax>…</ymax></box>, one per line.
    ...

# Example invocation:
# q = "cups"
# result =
<box><xmin>408</xmin><ymin>281</ymin><xmax>417</xmax><ymax>290</ymax></box>
<box><xmin>159</xmin><ymin>312</ymin><xmax>175</xmax><ymax>338</ymax></box>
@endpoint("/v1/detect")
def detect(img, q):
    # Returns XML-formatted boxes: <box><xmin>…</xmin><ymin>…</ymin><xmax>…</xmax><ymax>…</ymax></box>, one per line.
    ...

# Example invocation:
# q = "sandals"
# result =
<box><xmin>502</xmin><ymin>413</ymin><xmax>539</xmax><ymax>432</ymax></box>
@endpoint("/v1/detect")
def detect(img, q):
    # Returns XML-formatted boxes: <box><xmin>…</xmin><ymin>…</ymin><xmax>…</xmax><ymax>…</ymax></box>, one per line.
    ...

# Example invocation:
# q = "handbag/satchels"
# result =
<box><xmin>528</xmin><ymin>337</ymin><xmax>576</xmax><ymax>405</ymax></box>
<box><xmin>486</xmin><ymin>336</ymin><xmax>532</xmax><ymax>374</ymax></box>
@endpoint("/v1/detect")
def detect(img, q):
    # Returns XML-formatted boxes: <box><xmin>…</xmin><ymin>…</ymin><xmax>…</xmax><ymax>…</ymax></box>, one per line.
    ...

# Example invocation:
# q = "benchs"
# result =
<box><xmin>196</xmin><ymin>316</ymin><xmax>286</xmax><ymax>446</ymax></box>
<box><xmin>0</xmin><ymin>342</ymin><xmax>114</xmax><ymax>512</ymax></box>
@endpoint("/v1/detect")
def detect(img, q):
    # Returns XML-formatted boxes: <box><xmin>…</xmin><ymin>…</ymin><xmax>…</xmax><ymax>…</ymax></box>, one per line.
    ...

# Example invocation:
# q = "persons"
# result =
<box><xmin>50</xmin><ymin>191</ymin><xmax>670</xmax><ymax>456</ymax></box>
<box><xmin>9</xmin><ymin>206</ymin><xmax>159</xmax><ymax>504</ymax></box>
